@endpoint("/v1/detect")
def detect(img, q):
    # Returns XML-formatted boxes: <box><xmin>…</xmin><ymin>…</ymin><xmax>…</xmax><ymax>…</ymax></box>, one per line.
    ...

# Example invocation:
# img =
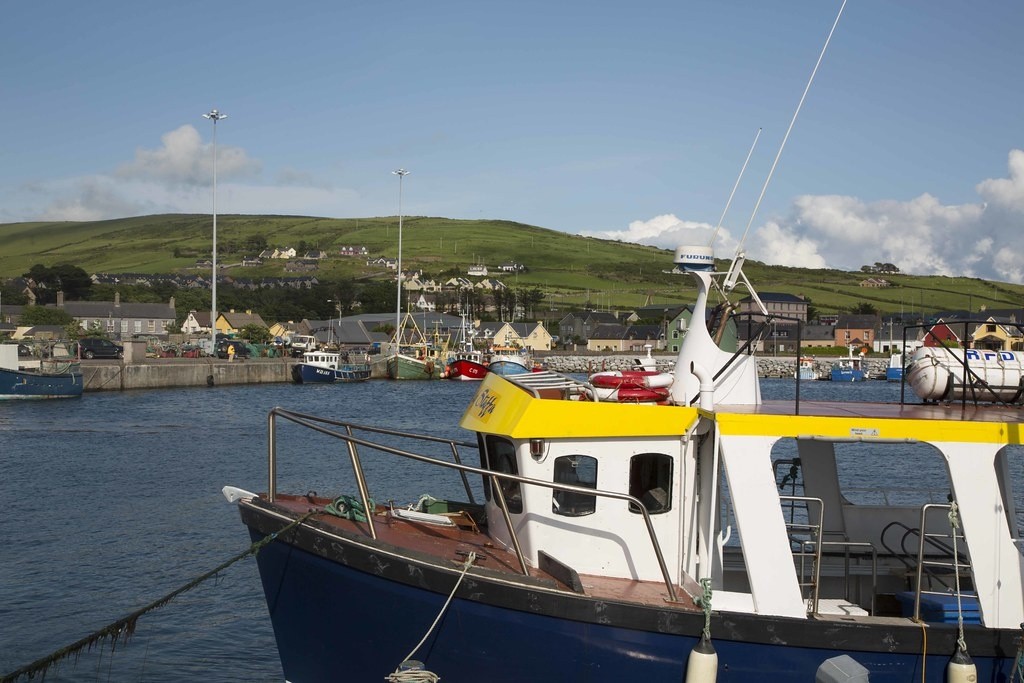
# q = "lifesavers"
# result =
<box><xmin>584</xmin><ymin>370</ymin><xmax>675</xmax><ymax>406</ymax></box>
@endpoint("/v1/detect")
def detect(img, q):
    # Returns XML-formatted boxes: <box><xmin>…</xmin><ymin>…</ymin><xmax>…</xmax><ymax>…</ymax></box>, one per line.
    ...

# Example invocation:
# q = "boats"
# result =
<box><xmin>297</xmin><ymin>349</ymin><xmax>374</xmax><ymax>385</ymax></box>
<box><xmin>0</xmin><ymin>342</ymin><xmax>85</xmax><ymax>402</ymax></box>
<box><xmin>793</xmin><ymin>353</ymin><xmax>824</xmax><ymax>381</ymax></box>
<box><xmin>885</xmin><ymin>352</ymin><xmax>908</xmax><ymax>384</ymax></box>
<box><xmin>386</xmin><ymin>296</ymin><xmax>535</xmax><ymax>383</ymax></box>
<box><xmin>828</xmin><ymin>344</ymin><xmax>869</xmax><ymax>383</ymax></box>
<box><xmin>224</xmin><ymin>2</ymin><xmax>1024</xmax><ymax>681</ymax></box>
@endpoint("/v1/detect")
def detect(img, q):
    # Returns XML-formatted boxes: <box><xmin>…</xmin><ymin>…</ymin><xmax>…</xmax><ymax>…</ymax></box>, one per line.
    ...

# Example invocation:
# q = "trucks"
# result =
<box><xmin>290</xmin><ymin>334</ymin><xmax>340</xmax><ymax>358</ymax></box>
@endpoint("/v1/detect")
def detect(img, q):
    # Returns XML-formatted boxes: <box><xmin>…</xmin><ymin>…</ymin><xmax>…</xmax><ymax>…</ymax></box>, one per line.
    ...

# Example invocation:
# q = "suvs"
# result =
<box><xmin>73</xmin><ymin>337</ymin><xmax>125</xmax><ymax>360</ymax></box>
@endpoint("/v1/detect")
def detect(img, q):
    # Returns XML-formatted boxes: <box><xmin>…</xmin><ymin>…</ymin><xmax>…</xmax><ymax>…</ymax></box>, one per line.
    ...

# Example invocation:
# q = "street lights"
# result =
<box><xmin>392</xmin><ymin>169</ymin><xmax>411</xmax><ymax>354</ymax></box>
<box><xmin>202</xmin><ymin>110</ymin><xmax>228</xmax><ymax>353</ymax></box>
<box><xmin>326</xmin><ymin>300</ymin><xmax>342</xmax><ymax>323</ymax></box>
